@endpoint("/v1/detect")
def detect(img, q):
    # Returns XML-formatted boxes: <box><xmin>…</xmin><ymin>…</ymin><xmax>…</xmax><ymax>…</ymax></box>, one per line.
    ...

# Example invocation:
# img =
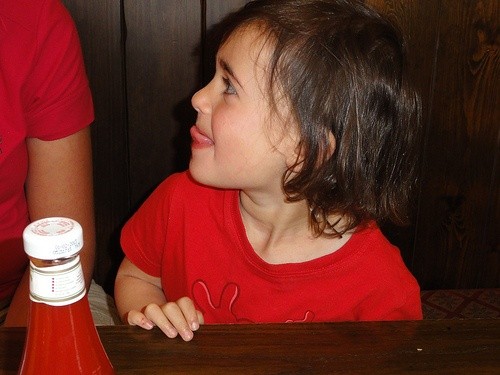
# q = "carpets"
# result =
<box><xmin>420</xmin><ymin>288</ymin><xmax>500</xmax><ymax>321</ymax></box>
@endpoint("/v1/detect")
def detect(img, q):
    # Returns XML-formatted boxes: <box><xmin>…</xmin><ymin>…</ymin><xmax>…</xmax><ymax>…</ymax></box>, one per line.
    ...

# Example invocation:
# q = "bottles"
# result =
<box><xmin>17</xmin><ymin>217</ymin><xmax>115</xmax><ymax>375</ymax></box>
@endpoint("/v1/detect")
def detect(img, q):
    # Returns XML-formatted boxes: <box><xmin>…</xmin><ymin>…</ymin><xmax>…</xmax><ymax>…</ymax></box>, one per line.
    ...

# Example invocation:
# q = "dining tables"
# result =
<box><xmin>1</xmin><ymin>317</ymin><xmax>500</xmax><ymax>373</ymax></box>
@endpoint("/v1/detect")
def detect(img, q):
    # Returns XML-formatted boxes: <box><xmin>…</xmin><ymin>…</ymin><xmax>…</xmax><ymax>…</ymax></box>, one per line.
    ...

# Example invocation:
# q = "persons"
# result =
<box><xmin>1</xmin><ymin>0</ymin><xmax>101</xmax><ymax>327</ymax></box>
<box><xmin>109</xmin><ymin>0</ymin><xmax>432</xmax><ymax>343</ymax></box>
